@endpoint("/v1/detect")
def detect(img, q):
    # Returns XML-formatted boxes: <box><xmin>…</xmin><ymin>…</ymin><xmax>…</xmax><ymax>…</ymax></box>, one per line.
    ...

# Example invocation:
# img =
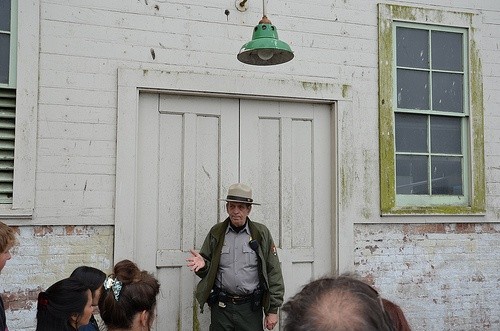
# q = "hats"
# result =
<box><xmin>217</xmin><ymin>184</ymin><xmax>261</xmax><ymax>205</ymax></box>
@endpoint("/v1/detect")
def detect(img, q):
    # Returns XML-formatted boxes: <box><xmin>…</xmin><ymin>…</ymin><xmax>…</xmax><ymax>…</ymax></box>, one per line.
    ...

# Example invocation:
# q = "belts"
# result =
<box><xmin>218</xmin><ymin>295</ymin><xmax>254</xmax><ymax>305</ymax></box>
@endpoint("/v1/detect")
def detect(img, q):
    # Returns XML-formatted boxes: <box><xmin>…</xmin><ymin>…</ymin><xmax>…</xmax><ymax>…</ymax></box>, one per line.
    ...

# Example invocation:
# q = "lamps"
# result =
<box><xmin>237</xmin><ymin>0</ymin><xmax>295</xmax><ymax>67</ymax></box>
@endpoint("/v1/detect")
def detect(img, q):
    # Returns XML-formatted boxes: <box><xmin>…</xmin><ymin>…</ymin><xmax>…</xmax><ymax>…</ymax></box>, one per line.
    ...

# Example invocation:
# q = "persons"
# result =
<box><xmin>0</xmin><ymin>220</ymin><xmax>19</xmax><ymax>331</ymax></box>
<box><xmin>69</xmin><ymin>266</ymin><xmax>107</xmax><ymax>331</ymax></box>
<box><xmin>98</xmin><ymin>259</ymin><xmax>160</xmax><ymax>331</ymax></box>
<box><xmin>280</xmin><ymin>272</ymin><xmax>412</xmax><ymax>331</ymax></box>
<box><xmin>185</xmin><ymin>183</ymin><xmax>285</xmax><ymax>331</ymax></box>
<box><xmin>35</xmin><ymin>278</ymin><xmax>94</xmax><ymax>331</ymax></box>
